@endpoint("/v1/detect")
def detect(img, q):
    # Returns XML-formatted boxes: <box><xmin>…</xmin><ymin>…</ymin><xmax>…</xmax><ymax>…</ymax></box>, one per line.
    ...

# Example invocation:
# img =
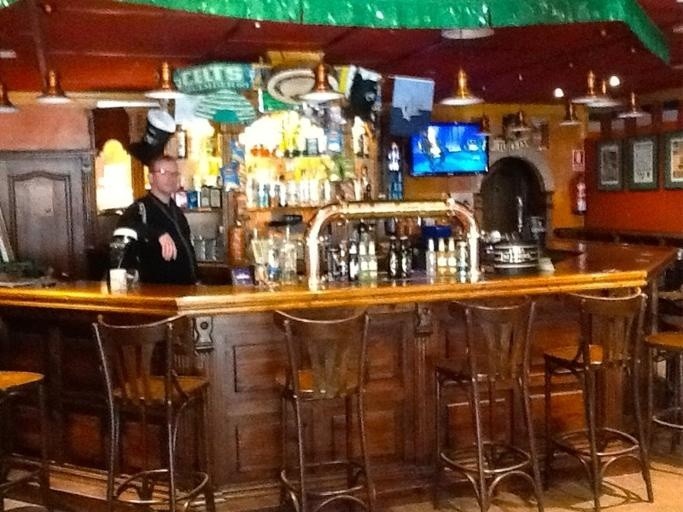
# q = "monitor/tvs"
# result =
<box><xmin>407</xmin><ymin>120</ymin><xmax>490</xmax><ymax>176</ymax></box>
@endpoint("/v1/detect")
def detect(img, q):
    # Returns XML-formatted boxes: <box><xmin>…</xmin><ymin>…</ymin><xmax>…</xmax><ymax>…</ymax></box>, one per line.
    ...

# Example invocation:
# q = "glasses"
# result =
<box><xmin>151</xmin><ymin>168</ymin><xmax>181</xmax><ymax>179</ymax></box>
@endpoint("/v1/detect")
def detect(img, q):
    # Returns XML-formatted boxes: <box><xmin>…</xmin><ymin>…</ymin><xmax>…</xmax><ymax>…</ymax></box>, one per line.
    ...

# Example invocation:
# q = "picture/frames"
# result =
<box><xmin>595</xmin><ymin>139</ymin><xmax>623</xmax><ymax>191</ymax></box>
<box><xmin>664</xmin><ymin>132</ymin><xmax>683</xmax><ymax>189</ymax></box>
<box><xmin>627</xmin><ymin>134</ymin><xmax>658</xmax><ymax>190</ymax></box>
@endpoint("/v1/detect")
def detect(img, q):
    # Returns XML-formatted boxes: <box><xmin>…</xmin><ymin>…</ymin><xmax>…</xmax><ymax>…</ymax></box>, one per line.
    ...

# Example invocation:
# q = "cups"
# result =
<box><xmin>110</xmin><ymin>269</ymin><xmax>126</xmax><ymax>291</ymax></box>
<box><xmin>193</xmin><ymin>239</ymin><xmax>216</xmax><ymax>261</ymax></box>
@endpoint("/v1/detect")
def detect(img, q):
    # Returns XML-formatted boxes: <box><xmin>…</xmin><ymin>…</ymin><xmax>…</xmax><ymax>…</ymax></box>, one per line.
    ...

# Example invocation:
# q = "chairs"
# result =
<box><xmin>274</xmin><ymin>306</ymin><xmax>376</xmax><ymax>512</ymax></box>
<box><xmin>1</xmin><ymin>369</ymin><xmax>51</xmax><ymax>512</ymax></box>
<box><xmin>543</xmin><ymin>286</ymin><xmax>648</xmax><ymax>511</ymax></box>
<box><xmin>92</xmin><ymin>313</ymin><xmax>217</xmax><ymax>512</ymax></box>
<box><xmin>645</xmin><ymin>279</ymin><xmax>683</xmax><ymax>474</ymax></box>
<box><xmin>432</xmin><ymin>295</ymin><xmax>544</xmax><ymax>512</ymax></box>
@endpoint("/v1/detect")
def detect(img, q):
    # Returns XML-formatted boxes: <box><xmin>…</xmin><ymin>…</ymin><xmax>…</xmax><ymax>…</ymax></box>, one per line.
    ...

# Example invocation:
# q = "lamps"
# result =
<box><xmin>560</xmin><ymin>70</ymin><xmax>645</xmax><ymax>125</ymax></box>
<box><xmin>0</xmin><ymin>81</ymin><xmax>18</xmax><ymax>114</ymax></box>
<box><xmin>511</xmin><ymin>108</ymin><xmax>533</xmax><ymax>132</ymax></box>
<box><xmin>443</xmin><ymin>29</ymin><xmax>495</xmax><ymax>40</ymax></box>
<box><xmin>441</xmin><ymin>29</ymin><xmax>485</xmax><ymax>106</ymax></box>
<box><xmin>34</xmin><ymin>66</ymin><xmax>70</xmax><ymax>104</ymax></box>
<box><xmin>298</xmin><ymin>52</ymin><xmax>344</xmax><ymax>103</ymax></box>
<box><xmin>144</xmin><ymin>57</ymin><xmax>185</xmax><ymax>99</ymax></box>
<box><xmin>475</xmin><ymin>113</ymin><xmax>495</xmax><ymax>136</ymax></box>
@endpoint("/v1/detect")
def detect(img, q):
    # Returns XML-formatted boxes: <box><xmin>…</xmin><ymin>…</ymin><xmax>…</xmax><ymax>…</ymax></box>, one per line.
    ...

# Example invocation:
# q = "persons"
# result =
<box><xmin>111</xmin><ymin>154</ymin><xmax>199</xmax><ymax>287</ymax></box>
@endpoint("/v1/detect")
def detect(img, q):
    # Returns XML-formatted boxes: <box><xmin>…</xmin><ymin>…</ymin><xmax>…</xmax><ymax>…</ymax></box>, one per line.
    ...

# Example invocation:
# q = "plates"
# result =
<box><xmin>0</xmin><ymin>279</ymin><xmax>38</xmax><ymax>288</ymax></box>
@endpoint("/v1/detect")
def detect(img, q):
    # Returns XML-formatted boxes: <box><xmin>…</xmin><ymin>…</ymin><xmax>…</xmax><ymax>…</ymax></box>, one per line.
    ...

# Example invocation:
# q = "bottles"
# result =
<box><xmin>175</xmin><ymin>115</ymin><xmax>370</xmax><ymax>208</ymax></box>
<box><xmin>217</xmin><ymin>216</ymin><xmax>458</xmax><ymax>285</ymax></box>
